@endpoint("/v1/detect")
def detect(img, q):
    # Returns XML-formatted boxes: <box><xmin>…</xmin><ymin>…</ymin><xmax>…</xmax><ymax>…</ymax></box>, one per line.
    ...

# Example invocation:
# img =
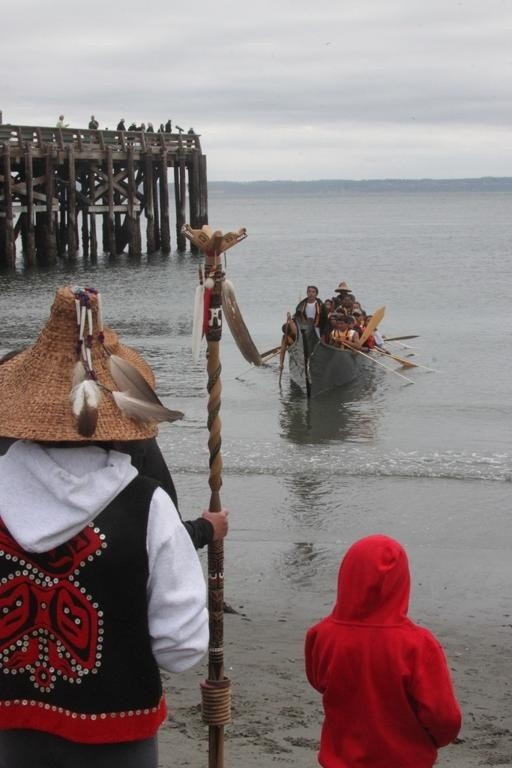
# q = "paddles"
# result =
<box><xmin>278</xmin><ymin>318</ymin><xmax>289</xmax><ymax>389</ymax></box>
<box><xmin>339</xmin><ymin>306</ymin><xmax>432</xmax><ymax>384</ymax></box>
<box><xmin>260</xmin><ymin>347</ymin><xmax>282</xmax><ymax>358</ymax></box>
<box><xmin>236</xmin><ymin>350</ymin><xmax>281</xmax><ymax>378</ymax></box>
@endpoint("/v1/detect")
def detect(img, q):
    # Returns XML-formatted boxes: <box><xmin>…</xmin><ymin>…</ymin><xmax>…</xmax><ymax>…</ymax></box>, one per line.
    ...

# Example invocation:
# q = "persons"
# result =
<box><xmin>116</xmin><ymin>118</ymin><xmax>195</xmax><ymax>151</ymax></box>
<box><xmin>304</xmin><ymin>534</ymin><xmax>461</xmax><ymax>768</ymax></box>
<box><xmin>291</xmin><ymin>282</ymin><xmax>385</xmax><ymax>352</ymax></box>
<box><xmin>1</xmin><ymin>344</ymin><xmax>228</xmax><ymax>554</ymax></box>
<box><xmin>56</xmin><ymin>115</ymin><xmax>69</xmax><ymax>128</ymax></box>
<box><xmin>1</xmin><ymin>285</ymin><xmax>208</xmax><ymax>768</ymax></box>
<box><xmin>89</xmin><ymin>115</ymin><xmax>99</xmax><ymax>130</ymax></box>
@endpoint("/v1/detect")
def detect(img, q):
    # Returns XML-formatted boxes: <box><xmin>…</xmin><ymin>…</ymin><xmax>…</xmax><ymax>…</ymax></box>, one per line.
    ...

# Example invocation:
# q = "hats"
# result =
<box><xmin>0</xmin><ymin>286</ymin><xmax>158</xmax><ymax>441</ymax></box>
<box><xmin>335</xmin><ymin>281</ymin><xmax>352</xmax><ymax>292</ymax></box>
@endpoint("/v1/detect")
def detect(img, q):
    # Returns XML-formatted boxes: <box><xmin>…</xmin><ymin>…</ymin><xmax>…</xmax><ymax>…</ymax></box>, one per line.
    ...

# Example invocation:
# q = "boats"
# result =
<box><xmin>286</xmin><ymin>317</ymin><xmax>371</xmax><ymax>400</ymax></box>
